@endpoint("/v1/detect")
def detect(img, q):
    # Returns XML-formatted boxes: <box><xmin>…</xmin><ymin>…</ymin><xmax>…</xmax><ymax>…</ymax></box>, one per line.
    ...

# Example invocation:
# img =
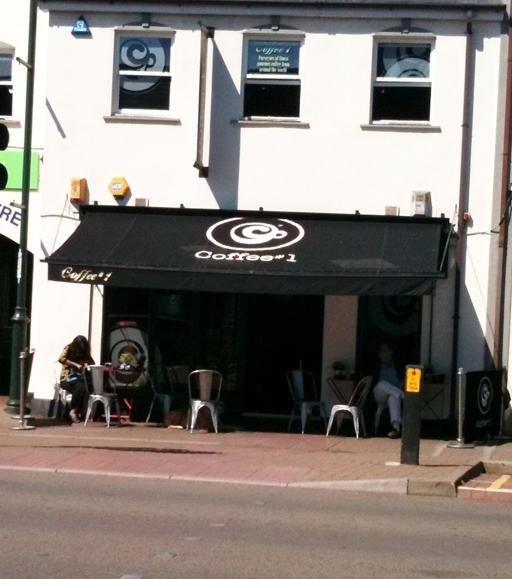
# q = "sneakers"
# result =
<box><xmin>387</xmin><ymin>427</ymin><xmax>400</xmax><ymax>438</ymax></box>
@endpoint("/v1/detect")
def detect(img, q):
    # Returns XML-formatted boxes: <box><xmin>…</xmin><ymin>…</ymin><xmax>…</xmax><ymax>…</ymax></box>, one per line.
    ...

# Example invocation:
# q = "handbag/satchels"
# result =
<box><xmin>66</xmin><ymin>366</ymin><xmax>91</xmax><ymax>384</ymax></box>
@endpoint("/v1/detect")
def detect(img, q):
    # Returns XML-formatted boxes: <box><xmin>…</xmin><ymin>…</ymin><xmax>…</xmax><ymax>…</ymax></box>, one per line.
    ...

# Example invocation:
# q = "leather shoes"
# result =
<box><xmin>68</xmin><ymin>413</ymin><xmax>80</xmax><ymax>423</ymax></box>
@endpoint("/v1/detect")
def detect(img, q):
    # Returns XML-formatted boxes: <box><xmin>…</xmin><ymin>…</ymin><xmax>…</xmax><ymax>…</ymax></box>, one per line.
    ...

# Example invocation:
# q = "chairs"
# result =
<box><xmin>283</xmin><ymin>368</ymin><xmax>375</xmax><ymax>440</ymax></box>
<box><xmin>82</xmin><ymin>364</ymin><xmax>223</xmax><ymax>436</ymax></box>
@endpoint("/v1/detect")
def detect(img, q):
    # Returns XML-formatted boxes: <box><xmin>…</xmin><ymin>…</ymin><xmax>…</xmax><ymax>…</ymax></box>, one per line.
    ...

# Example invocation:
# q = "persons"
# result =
<box><xmin>362</xmin><ymin>343</ymin><xmax>404</xmax><ymax>439</ymax></box>
<box><xmin>59</xmin><ymin>336</ymin><xmax>94</xmax><ymax>424</ymax></box>
